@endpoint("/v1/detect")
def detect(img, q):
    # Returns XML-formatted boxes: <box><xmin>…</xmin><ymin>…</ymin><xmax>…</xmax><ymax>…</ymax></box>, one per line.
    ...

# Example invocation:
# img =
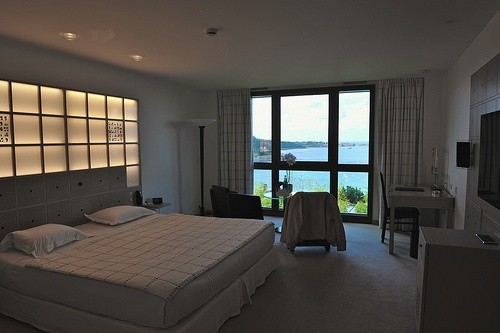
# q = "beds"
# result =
<box><xmin>0</xmin><ymin>164</ymin><xmax>280</xmax><ymax>333</ymax></box>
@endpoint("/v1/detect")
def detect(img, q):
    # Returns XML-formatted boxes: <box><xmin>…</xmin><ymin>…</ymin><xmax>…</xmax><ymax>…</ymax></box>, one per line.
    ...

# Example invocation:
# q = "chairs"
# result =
<box><xmin>379</xmin><ymin>172</ymin><xmax>421</xmax><ymax>257</ymax></box>
<box><xmin>210</xmin><ymin>185</ymin><xmax>264</xmax><ymax>220</ymax></box>
<box><xmin>278</xmin><ymin>191</ymin><xmax>347</xmax><ymax>254</ymax></box>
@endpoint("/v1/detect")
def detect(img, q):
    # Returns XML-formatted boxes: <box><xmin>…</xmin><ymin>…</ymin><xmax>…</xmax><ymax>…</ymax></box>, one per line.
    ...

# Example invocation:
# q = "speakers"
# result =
<box><xmin>456</xmin><ymin>142</ymin><xmax>471</xmax><ymax>168</ymax></box>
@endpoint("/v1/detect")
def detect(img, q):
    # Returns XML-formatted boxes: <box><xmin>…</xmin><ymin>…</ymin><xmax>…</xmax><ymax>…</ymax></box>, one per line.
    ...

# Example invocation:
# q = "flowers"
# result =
<box><xmin>282</xmin><ymin>153</ymin><xmax>297</xmax><ymax>184</ymax></box>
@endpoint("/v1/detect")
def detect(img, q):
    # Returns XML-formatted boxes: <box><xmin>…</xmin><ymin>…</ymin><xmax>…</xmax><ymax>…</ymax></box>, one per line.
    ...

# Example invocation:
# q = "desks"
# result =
<box><xmin>389</xmin><ymin>183</ymin><xmax>454</xmax><ymax>255</ymax></box>
<box><xmin>415</xmin><ymin>226</ymin><xmax>500</xmax><ymax>333</ymax></box>
<box><xmin>263</xmin><ymin>191</ymin><xmax>292</xmax><ymax>234</ymax></box>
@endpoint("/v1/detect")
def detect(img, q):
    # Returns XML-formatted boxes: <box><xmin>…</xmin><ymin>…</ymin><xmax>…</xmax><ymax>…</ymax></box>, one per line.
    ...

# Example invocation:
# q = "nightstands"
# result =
<box><xmin>139</xmin><ymin>202</ymin><xmax>172</xmax><ymax>214</ymax></box>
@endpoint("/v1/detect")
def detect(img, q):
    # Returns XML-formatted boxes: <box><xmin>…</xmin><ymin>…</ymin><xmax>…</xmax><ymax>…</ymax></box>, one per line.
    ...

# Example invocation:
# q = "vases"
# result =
<box><xmin>287</xmin><ymin>184</ymin><xmax>293</xmax><ymax>193</ymax></box>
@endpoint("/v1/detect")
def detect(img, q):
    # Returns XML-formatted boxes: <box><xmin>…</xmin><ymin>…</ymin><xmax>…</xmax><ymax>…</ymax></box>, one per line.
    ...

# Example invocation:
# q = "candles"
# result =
<box><xmin>432</xmin><ymin>147</ymin><xmax>438</xmax><ymax>167</ymax></box>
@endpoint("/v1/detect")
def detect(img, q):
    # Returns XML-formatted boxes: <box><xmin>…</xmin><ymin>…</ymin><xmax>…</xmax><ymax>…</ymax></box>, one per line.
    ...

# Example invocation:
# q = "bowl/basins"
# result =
<box><xmin>153</xmin><ymin>198</ymin><xmax>162</xmax><ymax>204</ymax></box>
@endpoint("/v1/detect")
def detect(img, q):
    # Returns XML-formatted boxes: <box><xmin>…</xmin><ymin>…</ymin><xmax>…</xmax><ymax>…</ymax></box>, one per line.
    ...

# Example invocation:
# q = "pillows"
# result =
<box><xmin>83</xmin><ymin>204</ymin><xmax>157</xmax><ymax>227</ymax></box>
<box><xmin>0</xmin><ymin>222</ymin><xmax>92</xmax><ymax>260</ymax></box>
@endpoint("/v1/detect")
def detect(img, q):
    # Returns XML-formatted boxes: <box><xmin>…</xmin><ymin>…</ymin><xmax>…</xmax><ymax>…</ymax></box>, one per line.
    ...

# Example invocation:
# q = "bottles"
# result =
<box><xmin>284</xmin><ymin>175</ymin><xmax>287</xmax><ymax>186</ymax></box>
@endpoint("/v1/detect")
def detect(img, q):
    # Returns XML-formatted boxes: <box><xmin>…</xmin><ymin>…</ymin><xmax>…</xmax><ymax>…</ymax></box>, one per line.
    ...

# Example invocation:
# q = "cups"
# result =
<box><xmin>288</xmin><ymin>184</ymin><xmax>292</xmax><ymax>192</ymax></box>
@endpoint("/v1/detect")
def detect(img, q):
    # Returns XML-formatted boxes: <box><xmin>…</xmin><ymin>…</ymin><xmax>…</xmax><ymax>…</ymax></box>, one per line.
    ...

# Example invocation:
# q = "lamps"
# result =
<box><xmin>188</xmin><ymin>117</ymin><xmax>216</xmax><ymax>217</ymax></box>
